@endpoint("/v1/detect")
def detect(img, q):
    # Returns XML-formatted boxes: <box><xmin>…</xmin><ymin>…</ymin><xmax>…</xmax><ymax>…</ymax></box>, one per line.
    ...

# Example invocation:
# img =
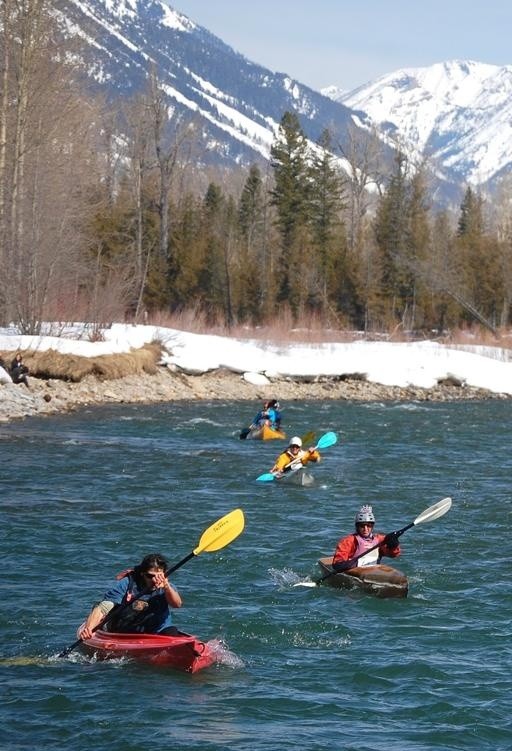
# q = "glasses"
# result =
<box><xmin>142</xmin><ymin>570</ymin><xmax>155</xmax><ymax>579</ymax></box>
<box><xmin>357</xmin><ymin>522</ymin><xmax>373</xmax><ymax>528</ymax></box>
<box><xmin>291</xmin><ymin>445</ymin><xmax>299</xmax><ymax>449</ymax></box>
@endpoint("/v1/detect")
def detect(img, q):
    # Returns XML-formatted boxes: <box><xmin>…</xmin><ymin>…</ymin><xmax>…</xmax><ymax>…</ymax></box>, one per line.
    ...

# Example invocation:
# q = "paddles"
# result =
<box><xmin>59</xmin><ymin>508</ymin><xmax>245</xmax><ymax>658</ymax></box>
<box><xmin>256</xmin><ymin>432</ymin><xmax>337</xmax><ymax>481</ymax></box>
<box><xmin>240</xmin><ymin>424</ymin><xmax>254</xmax><ymax>439</ymax></box>
<box><xmin>293</xmin><ymin>498</ymin><xmax>452</xmax><ymax>587</ymax></box>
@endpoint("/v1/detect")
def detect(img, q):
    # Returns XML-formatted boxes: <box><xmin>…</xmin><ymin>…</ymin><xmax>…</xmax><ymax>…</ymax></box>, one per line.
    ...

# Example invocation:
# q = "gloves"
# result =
<box><xmin>386</xmin><ymin>531</ymin><xmax>399</xmax><ymax>548</ymax></box>
<box><xmin>335</xmin><ymin>558</ymin><xmax>357</xmax><ymax>573</ymax></box>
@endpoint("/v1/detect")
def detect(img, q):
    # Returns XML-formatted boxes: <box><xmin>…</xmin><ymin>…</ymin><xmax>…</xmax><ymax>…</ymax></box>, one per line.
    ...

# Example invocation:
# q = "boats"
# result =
<box><xmin>280</xmin><ymin>468</ymin><xmax>314</xmax><ymax>486</ymax></box>
<box><xmin>316</xmin><ymin>554</ymin><xmax>409</xmax><ymax>599</ymax></box>
<box><xmin>75</xmin><ymin>619</ymin><xmax>218</xmax><ymax>675</ymax></box>
<box><xmin>245</xmin><ymin>419</ymin><xmax>285</xmax><ymax>441</ymax></box>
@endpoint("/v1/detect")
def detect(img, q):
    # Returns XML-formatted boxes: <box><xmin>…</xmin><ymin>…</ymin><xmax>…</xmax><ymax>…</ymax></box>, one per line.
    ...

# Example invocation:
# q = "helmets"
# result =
<box><xmin>356</xmin><ymin>504</ymin><xmax>376</xmax><ymax>525</ymax></box>
<box><xmin>289</xmin><ymin>437</ymin><xmax>302</xmax><ymax>448</ymax></box>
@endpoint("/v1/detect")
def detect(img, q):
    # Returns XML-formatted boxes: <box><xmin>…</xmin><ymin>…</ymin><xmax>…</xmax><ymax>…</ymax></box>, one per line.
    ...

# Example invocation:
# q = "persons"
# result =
<box><xmin>271</xmin><ymin>436</ymin><xmax>321</xmax><ymax>478</ymax></box>
<box><xmin>332</xmin><ymin>507</ymin><xmax>407</xmax><ymax>585</ymax></box>
<box><xmin>240</xmin><ymin>399</ymin><xmax>281</xmax><ymax>441</ymax></box>
<box><xmin>79</xmin><ymin>554</ymin><xmax>183</xmax><ymax>641</ymax></box>
